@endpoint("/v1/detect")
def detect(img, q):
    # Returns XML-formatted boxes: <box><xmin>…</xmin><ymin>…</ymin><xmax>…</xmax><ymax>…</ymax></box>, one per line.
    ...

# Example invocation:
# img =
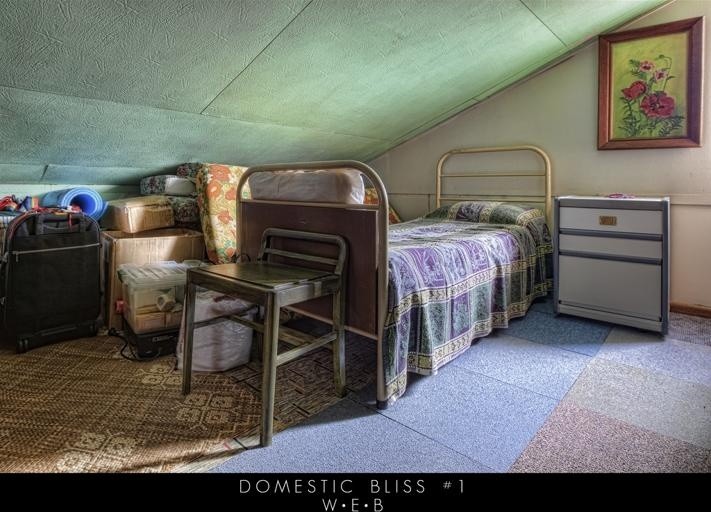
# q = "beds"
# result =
<box><xmin>236</xmin><ymin>145</ymin><xmax>554</xmax><ymax>408</ymax></box>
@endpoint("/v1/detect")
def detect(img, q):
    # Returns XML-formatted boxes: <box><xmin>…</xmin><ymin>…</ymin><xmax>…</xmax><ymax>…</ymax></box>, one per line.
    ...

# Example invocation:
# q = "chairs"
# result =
<box><xmin>182</xmin><ymin>228</ymin><xmax>351</xmax><ymax>446</ymax></box>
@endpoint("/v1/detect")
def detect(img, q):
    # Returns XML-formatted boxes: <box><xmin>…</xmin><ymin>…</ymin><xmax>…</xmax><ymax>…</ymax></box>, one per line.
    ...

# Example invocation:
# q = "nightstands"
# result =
<box><xmin>555</xmin><ymin>196</ymin><xmax>671</xmax><ymax>335</ymax></box>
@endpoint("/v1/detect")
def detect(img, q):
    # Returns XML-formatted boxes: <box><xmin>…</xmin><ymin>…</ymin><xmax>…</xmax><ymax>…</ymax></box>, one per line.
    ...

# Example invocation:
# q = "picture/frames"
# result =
<box><xmin>598</xmin><ymin>16</ymin><xmax>706</xmax><ymax>150</ymax></box>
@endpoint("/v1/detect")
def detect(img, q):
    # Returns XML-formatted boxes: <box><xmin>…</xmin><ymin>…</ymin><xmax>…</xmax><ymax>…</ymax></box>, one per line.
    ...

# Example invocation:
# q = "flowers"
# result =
<box><xmin>619</xmin><ymin>53</ymin><xmax>687</xmax><ymax>139</ymax></box>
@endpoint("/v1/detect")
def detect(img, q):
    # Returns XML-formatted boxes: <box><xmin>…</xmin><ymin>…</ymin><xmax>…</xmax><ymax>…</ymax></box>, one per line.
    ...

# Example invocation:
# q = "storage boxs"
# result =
<box><xmin>102</xmin><ymin>228</ymin><xmax>205</xmax><ymax>329</ymax></box>
<box><xmin>101</xmin><ymin>196</ymin><xmax>175</xmax><ymax>233</ymax></box>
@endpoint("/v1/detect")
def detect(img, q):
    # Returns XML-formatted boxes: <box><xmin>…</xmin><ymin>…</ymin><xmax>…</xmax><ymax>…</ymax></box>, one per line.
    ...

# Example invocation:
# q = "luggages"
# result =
<box><xmin>1</xmin><ymin>209</ymin><xmax>103</xmax><ymax>354</ymax></box>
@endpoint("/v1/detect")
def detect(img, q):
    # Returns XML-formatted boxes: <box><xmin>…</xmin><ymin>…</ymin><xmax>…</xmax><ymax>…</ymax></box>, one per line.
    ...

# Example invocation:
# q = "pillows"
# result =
<box><xmin>425</xmin><ymin>201</ymin><xmax>544</xmax><ymax>226</ymax></box>
